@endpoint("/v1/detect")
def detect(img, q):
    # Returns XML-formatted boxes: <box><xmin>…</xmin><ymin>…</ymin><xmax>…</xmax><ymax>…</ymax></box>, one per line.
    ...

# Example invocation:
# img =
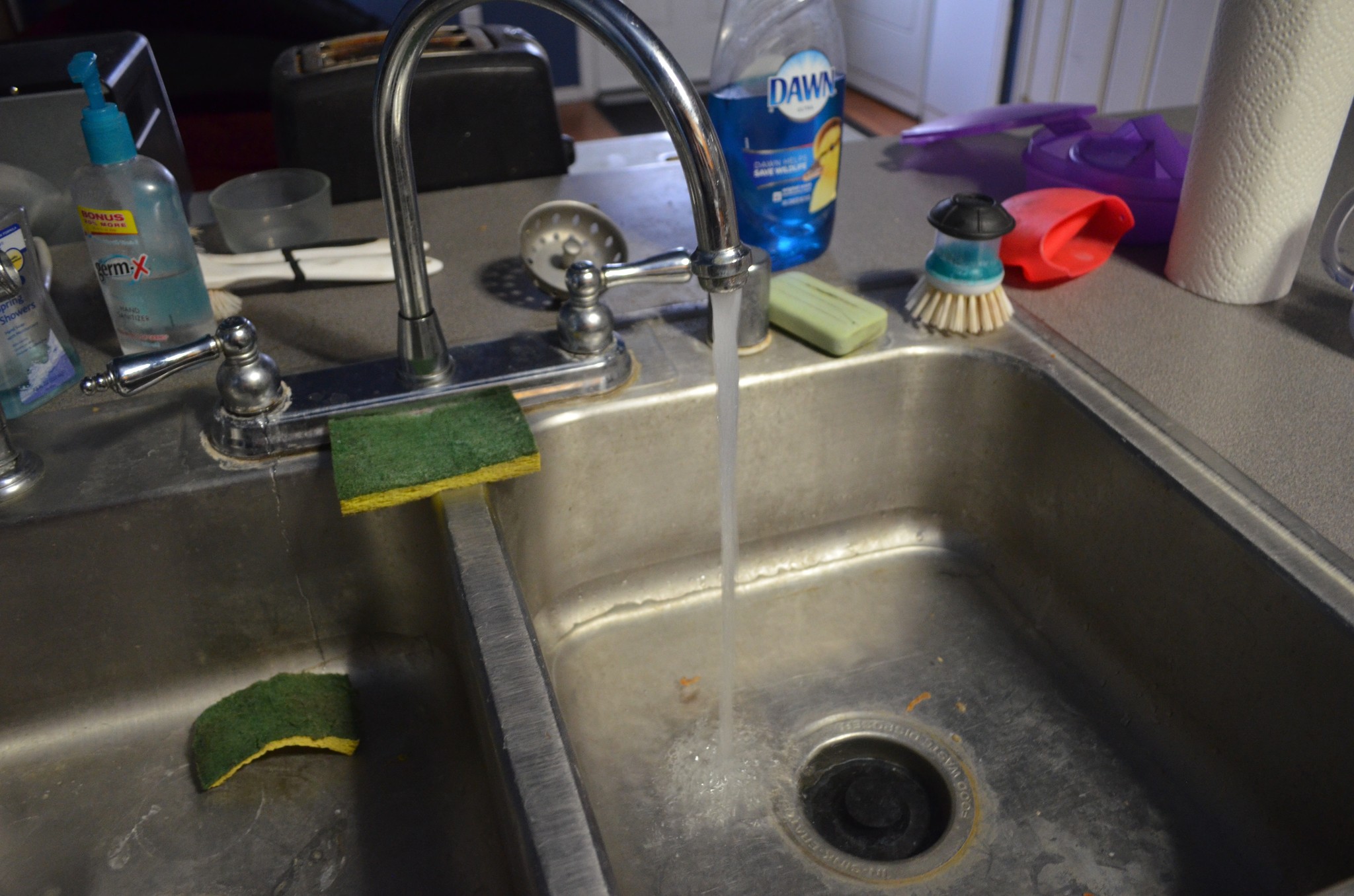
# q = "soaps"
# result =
<box><xmin>764</xmin><ymin>268</ymin><xmax>888</xmax><ymax>358</ymax></box>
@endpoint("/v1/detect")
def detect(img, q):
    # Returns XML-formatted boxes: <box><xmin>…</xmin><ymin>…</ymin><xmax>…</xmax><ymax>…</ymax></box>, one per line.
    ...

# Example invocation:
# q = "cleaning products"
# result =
<box><xmin>66</xmin><ymin>49</ymin><xmax>220</xmax><ymax>365</ymax></box>
<box><xmin>0</xmin><ymin>177</ymin><xmax>87</xmax><ymax>423</ymax></box>
<box><xmin>705</xmin><ymin>0</ymin><xmax>847</xmax><ymax>270</ymax></box>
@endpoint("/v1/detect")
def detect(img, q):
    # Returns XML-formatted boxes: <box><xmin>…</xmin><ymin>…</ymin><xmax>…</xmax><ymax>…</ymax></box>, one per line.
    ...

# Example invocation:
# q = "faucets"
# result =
<box><xmin>372</xmin><ymin>0</ymin><xmax>754</xmax><ymax>383</ymax></box>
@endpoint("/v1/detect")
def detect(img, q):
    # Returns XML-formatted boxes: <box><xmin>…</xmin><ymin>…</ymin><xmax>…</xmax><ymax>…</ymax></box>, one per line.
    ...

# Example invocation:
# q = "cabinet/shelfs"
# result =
<box><xmin>0</xmin><ymin>34</ymin><xmax>186</xmax><ymax>211</ymax></box>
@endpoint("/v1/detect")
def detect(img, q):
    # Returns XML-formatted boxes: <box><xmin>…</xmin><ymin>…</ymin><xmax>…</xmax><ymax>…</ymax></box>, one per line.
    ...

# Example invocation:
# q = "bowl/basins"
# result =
<box><xmin>210</xmin><ymin>167</ymin><xmax>332</xmax><ymax>251</ymax></box>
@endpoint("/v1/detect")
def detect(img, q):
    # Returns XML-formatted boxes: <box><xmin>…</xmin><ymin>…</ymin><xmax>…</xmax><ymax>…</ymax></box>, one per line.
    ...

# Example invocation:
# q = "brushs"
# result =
<box><xmin>902</xmin><ymin>192</ymin><xmax>1017</xmax><ymax>337</ymax></box>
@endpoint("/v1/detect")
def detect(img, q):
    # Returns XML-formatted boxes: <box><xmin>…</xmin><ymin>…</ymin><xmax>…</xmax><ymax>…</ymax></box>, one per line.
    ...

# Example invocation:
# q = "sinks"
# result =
<box><xmin>4</xmin><ymin>426</ymin><xmax>606</xmax><ymax>892</ymax></box>
<box><xmin>448</xmin><ymin>278</ymin><xmax>1354</xmax><ymax>894</ymax></box>
